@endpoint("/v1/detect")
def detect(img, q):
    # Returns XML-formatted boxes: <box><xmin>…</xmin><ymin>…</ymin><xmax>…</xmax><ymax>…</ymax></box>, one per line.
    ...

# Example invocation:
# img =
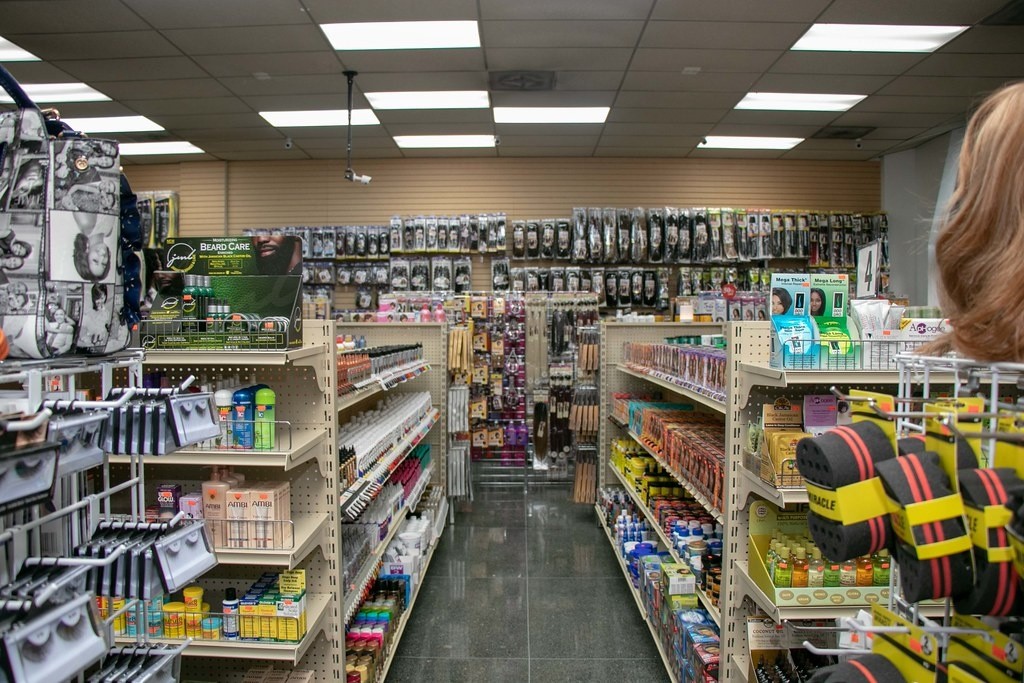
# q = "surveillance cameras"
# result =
<box><xmin>284</xmin><ymin>138</ymin><xmax>292</xmax><ymax>149</ymax></box>
<box><xmin>854</xmin><ymin>139</ymin><xmax>864</xmax><ymax>150</ymax></box>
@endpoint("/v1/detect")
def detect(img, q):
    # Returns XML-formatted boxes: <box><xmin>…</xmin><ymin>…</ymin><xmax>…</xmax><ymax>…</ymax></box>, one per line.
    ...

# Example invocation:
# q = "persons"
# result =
<box><xmin>387</xmin><ymin>315</ymin><xmax>393</xmax><ymax>322</ymax></box>
<box><xmin>250</xmin><ymin>236</ymin><xmax>302</xmax><ymax>276</ymax></box>
<box><xmin>733</xmin><ymin>309</ymin><xmax>739</xmax><ymax>318</ymax></box>
<box><xmin>837</xmin><ymin>402</ymin><xmax>849</xmax><ymax>421</ymax></box>
<box><xmin>745</xmin><ymin>310</ymin><xmax>753</xmax><ymax>320</ymax></box>
<box><xmin>365</xmin><ymin>314</ymin><xmax>374</xmax><ymax>322</ymax></box>
<box><xmin>0</xmin><ymin>110</ymin><xmax>131</xmax><ymax>357</ymax></box>
<box><xmin>399</xmin><ymin>314</ymin><xmax>408</xmax><ymax>322</ymax></box>
<box><xmin>353</xmin><ymin>314</ymin><xmax>363</xmax><ymax>322</ymax></box>
<box><xmin>772</xmin><ymin>287</ymin><xmax>792</xmax><ymax>315</ymax></box>
<box><xmin>757</xmin><ymin>310</ymin><xmax>765</xmax><ymax>320</ymax></box>
<box><xmin>911</xmin><ymin>80</ymin><xmax>1024</xmax><ymax>363</ymax></box>
<box><xmin>336</xmin><ymin>314</ymin><xmax>343</xmax><ymax>322</ymax></box>
<box><xmin>154</xmin><ymin>272</ymin><xmax>183</xmax><ymax>295</ymax></box>
<box><xmin>811</xmin><ymin>288</ymin><xmax>826</xmax><ymax>316</ymax></box>
<box><xmin>390</xmin><ymin>302</ymin><xmax>396</xmax><ymax>310</ymax></box>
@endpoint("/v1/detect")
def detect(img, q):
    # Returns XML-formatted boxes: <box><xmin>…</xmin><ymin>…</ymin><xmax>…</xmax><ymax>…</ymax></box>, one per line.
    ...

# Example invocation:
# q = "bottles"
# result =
<box><xmin>766</xmin><ymin>527</ymin><xmax>890</xmax><ymax>588</ymax></box>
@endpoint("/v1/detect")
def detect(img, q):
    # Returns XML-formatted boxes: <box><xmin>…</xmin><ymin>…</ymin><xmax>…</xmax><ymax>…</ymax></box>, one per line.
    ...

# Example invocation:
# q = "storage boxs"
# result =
<box><xmin>761</xmin><ymin>397</ymin><xmax>806</xmax><ymax>489</ymax></box>
<box><xmin>670</xmin><ymin>291</ymin><xmax>767</xmax><ymax>322</ymax></box>
<box><xmin>769</xmin><ymin>273</ymin><xmax>950</xmax><ymax>371</ymax></box>
<box><xmin>748</xmin><ymin>648</ymin><xmax>840</xmax><ymax>683</ymax></box>
<box><xmin>226</xmin><ymin>479</ymin><xmax>292</xmax><ymax>548</ymax></box>
<box><xmin>138</xmin><ymin>235</ymin><xmax>303</xmax><ymax>350</ymax></box>
<box><xmin>638</xmin><ymin>553</ymin><xmax>719</xmax><ymax>683</ymax></box>
<box><xmin>240</xmin><ymin>571</ymin><xmax>308</xmax><ymax>643</ymax></box>
<box><xmin>137</xmin><ymin>483</ymin><xmax>204</xmax><ymax>519</ymax></box>
<box><xmin>749</xmin><ymin>501</ymin><xmax>898</xmax><ymax>607</ymax></box>
<box><xmin>243</xmin><ymin>663</ymin><xmax>316</xmax><ymax>683</ymax></box>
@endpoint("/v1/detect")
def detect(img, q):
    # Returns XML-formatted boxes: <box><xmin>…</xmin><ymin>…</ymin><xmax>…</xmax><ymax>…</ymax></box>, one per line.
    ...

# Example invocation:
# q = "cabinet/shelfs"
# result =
<box><xmin>594</xmin><ymin>320</ymin><xmax>1017</xmax><ymax>683</ymax></box>
<box><xmin>99</xmin><ymin>319</ymin><xmax>454</xmax><ymax>683</ymax></box>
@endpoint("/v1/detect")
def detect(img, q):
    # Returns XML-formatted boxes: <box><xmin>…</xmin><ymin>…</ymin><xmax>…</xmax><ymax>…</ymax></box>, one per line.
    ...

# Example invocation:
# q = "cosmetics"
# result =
<box><xmin>158</xmin><ymin>374</ymin><xmax>275</xmax><ymax>451</ymax></box>
<box><xmin>226</xmin><ymin>480</ymin><xmax>291</xmax><ymax>549</ymax></box>
<box><xmin>184</xmin><ymin>274</ymin><xmax>288</xmax><ymax>332</ymax></box>
